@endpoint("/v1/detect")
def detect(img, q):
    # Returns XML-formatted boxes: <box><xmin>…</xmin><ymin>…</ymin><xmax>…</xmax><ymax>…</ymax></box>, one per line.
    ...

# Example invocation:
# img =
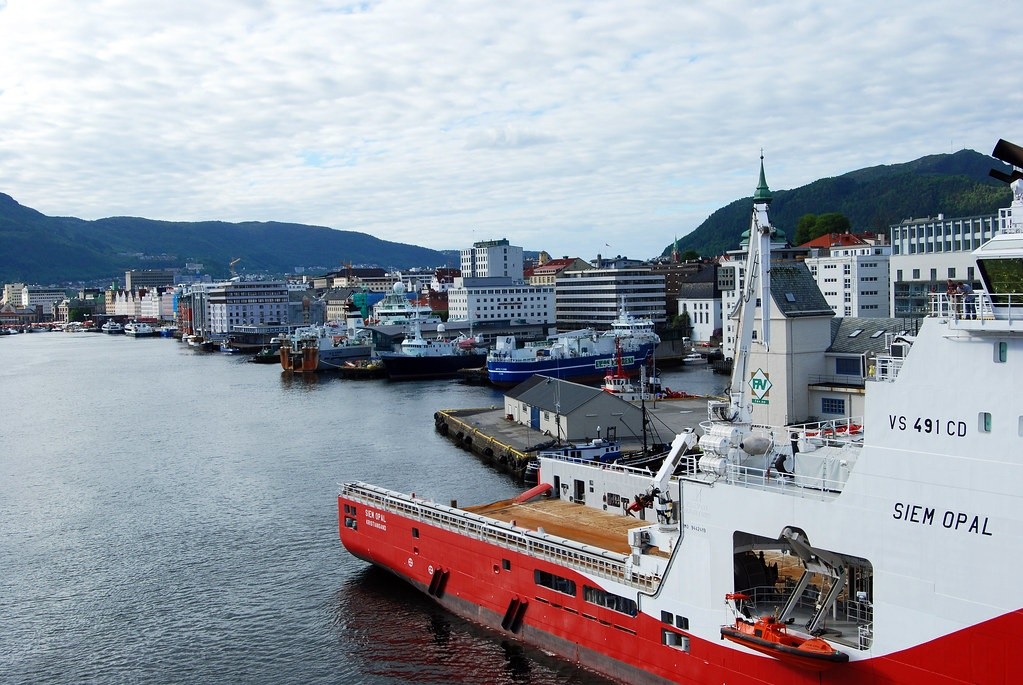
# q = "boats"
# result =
<box><xmin>378</xmin><ymin>293</ymin><xmax>489</xmax><ymax>378</ymax></box>
<box><xmin>682</xmin><ymin>347</ymin><xmax>708</xmax><ymax>366</ymax></box>
<box><xmin>101</xmin><ymin>318</ymin><xmax>124</xmax><ymax>334</ymax></box>
<box><xmin>246</xmin><ymin>320</ymin><xmax>387</xmax><ymax>380</ymax></box>
<box><xmin>124</xmin><ymin>321</ymin><xmax>157</xmax><ymax>338</ymax></box>
<box><xmin>514</xmin><ymin>482</ymin><xmax>553</xmax><ymax>504</ymax></box>
<box><xmin>626</xmin><ymin>496</ymin><xmax>650</xmax><ymax>512</ymax></box>
<box><xmin>600</xmin><ymin>335</ymin><xmax>634</xmax><ymax>393</ymax></box>
<box><xmin>525</xmin><ymin>355</ymin><xmax>624</xmax><ymax>488</ymax></box>
<box><xmin>719</xmin><ymin>593</ymin><xmax>850</xmax><ymax>674</ymax></box>
<box><xmin>486</xmin><ymin>291</ymin><xmax>661</xmax><ymax>388</ymax></box>
<box><xmin>334</xmin><ymin>204</ymin><xmax>1023</xmax><ymax>685</ymax></box>
<box><xmin>0</xmin><ymin>319</ymin><xmax>102</xmax><ymax>336</ymax></box>
<box><xmin>182</xmin><ymin>332</ymin><xmax>241</xmax><ymax>354</ymax></box>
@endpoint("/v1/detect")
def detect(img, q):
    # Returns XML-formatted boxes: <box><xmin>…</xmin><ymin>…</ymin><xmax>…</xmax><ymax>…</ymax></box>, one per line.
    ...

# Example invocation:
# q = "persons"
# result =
<box><xmin>957</xmin><ymin>282</ymin><xmax>977</xmax><ymax>319</ymax></box>
<box><xmin>946</xmin><ymin>280</ymin><xmax>961</xmax><ymax>319</ymax></box>
